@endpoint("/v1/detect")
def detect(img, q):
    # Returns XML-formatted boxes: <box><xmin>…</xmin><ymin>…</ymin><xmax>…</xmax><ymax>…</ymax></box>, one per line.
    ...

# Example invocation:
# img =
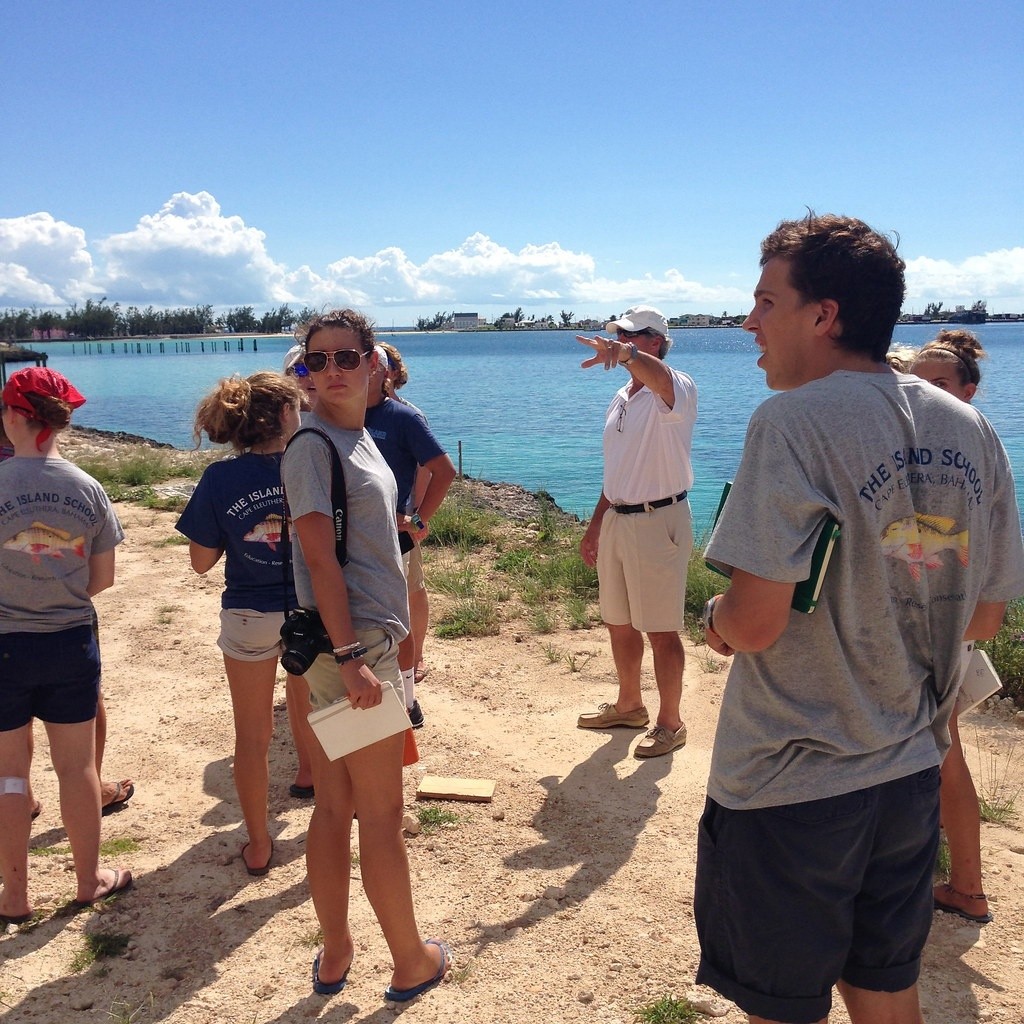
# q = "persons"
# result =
<box><xmin>174</xmin><ymin>343</ymin><xmax>319</xmax><ymax>876</ymax></box>
<box><xmin>0</xmin><ymin>367</ymin><xmax>135</xmax><ymax>924</ymax></box>
<box><xmin>887</xmin><ymin>327</ymin><xmax>993</xmax><ymax>923</ymax></box>
<box><xmin>280</xmin><ymin>309</ymin><xmax>452</xmax><ymax>1002</ymax></box>
<box><xmin>575</xmin><ymin>303</ymin><xmax>699</xmax><ymax>757</ymax></box>
<box><xmin>363</xmin><ymin>346</ymin><xmax>456</xmax><ymax>728</ymax></box>
<box><xmin>694</xmin><ymin>212</ymin><xmax>1024</xmax><ymax>1024</ymax></box>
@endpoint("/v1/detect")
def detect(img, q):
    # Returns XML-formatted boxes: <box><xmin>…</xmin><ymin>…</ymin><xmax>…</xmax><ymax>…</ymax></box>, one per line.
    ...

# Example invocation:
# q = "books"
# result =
<box><xmin>306</xmin><ymin>681</ymin><xmax>413</xmax><ymax>762</ymax></box>
<box><xmin>706</xmin><ymin>481</ymin><xmax>839</xmax><ymax>614</ymax></box>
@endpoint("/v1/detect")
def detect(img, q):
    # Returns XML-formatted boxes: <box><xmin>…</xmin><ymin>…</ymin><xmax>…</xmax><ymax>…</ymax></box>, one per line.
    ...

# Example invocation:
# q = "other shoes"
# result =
<box><xmin>408</xmin><ymin>702</ymin><xmax>425</xmax><ymax>727</ymax></box>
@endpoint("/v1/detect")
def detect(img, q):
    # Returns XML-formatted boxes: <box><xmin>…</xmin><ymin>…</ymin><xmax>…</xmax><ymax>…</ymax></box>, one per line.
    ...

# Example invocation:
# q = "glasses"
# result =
<box><xmin>287</xmin><ymin>363</ymin><xmax>309</xmax><ymax>378</ymax></box>
<box><xmin>616</xmin><ymin>328</ymin><xmax>655</xmax><ymax>339</ymax></box>
<box><xmin>302</xmin><ymin>350</ymin><xmax>374</xmax><ymax>373</ymax></box>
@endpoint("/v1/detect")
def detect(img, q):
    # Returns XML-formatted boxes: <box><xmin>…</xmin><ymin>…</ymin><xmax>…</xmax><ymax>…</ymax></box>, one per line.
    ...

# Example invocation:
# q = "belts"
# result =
<box><xmin>610</xmin><ymin>490</ymin><xmax>688</xmax><ymax>514</ymax></box>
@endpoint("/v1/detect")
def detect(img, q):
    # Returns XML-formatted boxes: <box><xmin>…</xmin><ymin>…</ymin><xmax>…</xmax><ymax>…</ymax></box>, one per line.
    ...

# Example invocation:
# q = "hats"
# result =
<box><xmin>606</xmin><ymin>304</ymin><xmax>668</xmax><ymax>340</ymax></box>
<box><xmin>283</xmin><ymin>342</ymin><xmax>306</xmax><ymax>376</ymax></box>
<box><xmin>374</xmin><ymin>345</ymin><xmax>389</xmax><ymax>371</ymax></box>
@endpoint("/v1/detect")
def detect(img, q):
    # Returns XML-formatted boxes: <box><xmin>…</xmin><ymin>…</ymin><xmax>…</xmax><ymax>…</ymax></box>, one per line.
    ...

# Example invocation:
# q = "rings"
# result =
<box><xmin>607</xmin><ymin>344</ymin><xmax>613</xmax><ymax>350</ymax></box>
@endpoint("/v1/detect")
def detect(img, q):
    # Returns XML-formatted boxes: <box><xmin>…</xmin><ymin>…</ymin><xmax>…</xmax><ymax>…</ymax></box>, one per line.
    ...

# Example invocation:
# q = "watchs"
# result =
<box><xmin>411</xmin><ymin>514</ymin><xmax>424</xmax><ymax>530</ymax></box>
<box><xmin>703</xmin><ymin>595</ymin><xmax>721</xmax><ymax>635</ymax></box>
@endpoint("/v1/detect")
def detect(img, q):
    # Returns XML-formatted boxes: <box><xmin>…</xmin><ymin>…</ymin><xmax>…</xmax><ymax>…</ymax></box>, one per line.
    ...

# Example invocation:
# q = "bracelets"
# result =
<box><xmin>332</xmin><ymin>642</ymin><xmax>360</xmax><ymax>653</ymax></box>
<box><xmin>618</xmin><ymin>342</ymin><xmax>637</xmax><ymax>366</ymax></box>
<box><xmin>335</xmin><ymin>647</ymin><xmax>368</xmax><ymax>665</ymax></box>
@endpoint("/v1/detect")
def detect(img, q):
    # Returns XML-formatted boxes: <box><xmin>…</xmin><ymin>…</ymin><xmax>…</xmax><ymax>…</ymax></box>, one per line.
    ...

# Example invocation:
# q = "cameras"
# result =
<box><xmin>280</xmin><ymin>608</ymin><xmax>332</xmax><ymax>676</ymax></box>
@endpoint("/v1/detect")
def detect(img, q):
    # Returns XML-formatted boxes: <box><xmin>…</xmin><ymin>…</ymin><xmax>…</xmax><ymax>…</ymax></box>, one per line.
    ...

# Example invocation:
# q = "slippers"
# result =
<box><xmin>240</xmin><ymin>834</ymin><xmax>274</xmax><ymax>875</ymax></box>
<box><xmin>31</xmin><ymin>801</ymin><xmax>43</xmax><ymax>820</ymax></box>
<box><xmin>386</xmin><ymin>937</ymin><xmax>451</xmax><ymax>1001</ymax></box>
<box><xmin>287</xmin><ymin>783</ymin><xmax>316</xmax><ymax>798</ymax></box>
<box><xmin>103</xmin><ymin>781</ymin><xmax>134</xmax><ymax>812</ymax></box>
<box><xmin>934</xmin><ymin>888</ymin><xmax>993</xmax><ymax>923</ymax></box>
<box><xmin>313</xmin><ymin>948</ymin><xmax>350</xmax><ymax>994</ymax></box>
<box><xmin>413</xmin><ymin>667</ymin><xmax>430</xmax><ymax>683</ymax></box>
<box><xmin>0</xmin><ymin>911</ymin><xmax>34</xmax><ymax>924</ymax></box>
<box><xmin>72</xmin><ymin>869</ymin><xmax>133</xmax><ymax>907</ymax></box>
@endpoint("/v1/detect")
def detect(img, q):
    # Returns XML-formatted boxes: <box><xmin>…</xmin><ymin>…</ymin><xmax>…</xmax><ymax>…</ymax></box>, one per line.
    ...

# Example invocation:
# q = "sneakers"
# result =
<box><xmin>635</xmin><ymin>721</ymin><xmax>686</xmax><ymax>756</ymax></box>
<box><xmin>579</xmin><ymin>702</ymin><xmax>651</xmax><ymax>728</ymax></box>
